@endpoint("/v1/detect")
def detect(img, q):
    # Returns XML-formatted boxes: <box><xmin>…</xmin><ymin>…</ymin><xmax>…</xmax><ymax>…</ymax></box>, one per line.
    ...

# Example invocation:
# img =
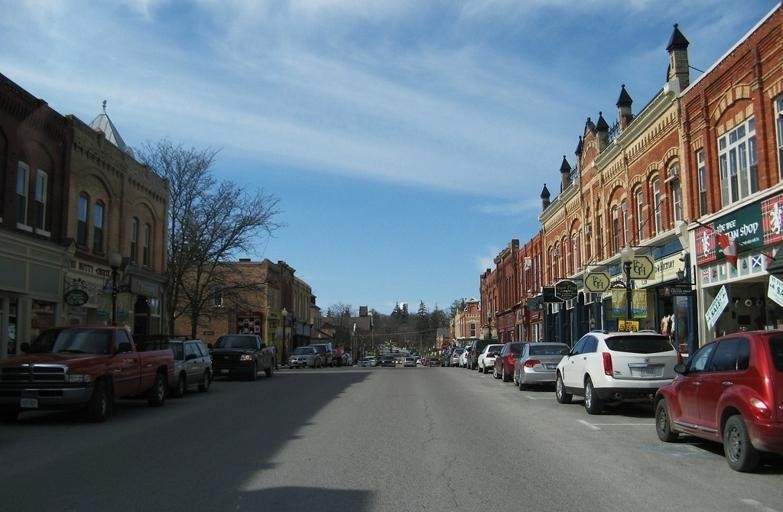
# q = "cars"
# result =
<box><xmin>442</xmin><ymin>339</ymin><xmax>572</xmax><ymax>391</ymax></box>
<box><xmin>341</xmin><ymin>350</ymin><xmax>440</xmax><ymax>367</ymax></box>
<box><xmin>651</xmin><ymin>328</ymin><xmax>783</xmax><ymax>474</ymax></box>
<box><xmin>287</xmin><ymin>346</ymin><xmax>323</xmax><ymax>369</ymax></box>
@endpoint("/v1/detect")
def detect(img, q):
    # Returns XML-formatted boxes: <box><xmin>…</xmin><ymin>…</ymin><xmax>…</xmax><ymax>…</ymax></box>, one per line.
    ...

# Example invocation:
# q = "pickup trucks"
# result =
<box><xmin>1</xmin><ymin>322</ymin><xmax>178</xmax><ymax>426</ymax></box>
<box><xmin>207</xmin><ymin>332</ymin><xmax>278</xmax><ymax>382</ymax></box>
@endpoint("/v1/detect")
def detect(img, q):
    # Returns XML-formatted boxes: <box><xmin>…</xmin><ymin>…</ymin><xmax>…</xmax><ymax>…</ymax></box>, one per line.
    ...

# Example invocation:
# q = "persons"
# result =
<box><xmin>332</xmin><ymin>344</ymin><xmax>343</xmax><ymax>368</ymax></box>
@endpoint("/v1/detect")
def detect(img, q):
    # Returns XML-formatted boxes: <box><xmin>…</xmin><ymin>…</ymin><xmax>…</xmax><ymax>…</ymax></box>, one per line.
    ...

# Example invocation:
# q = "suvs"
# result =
<box><xmin>307</xmin><ymin>343</ymin><xmax>334</xmax><ymax>367</ymax></box>
<box><xmin>145</xmin><ymin>333</ymin><xmax>214</xmax><ymax>399</ymax></box>
<box><xmin>552</xmin><ymin>327</ymin><xmax>685</xmax><ymax>416</ymax></box>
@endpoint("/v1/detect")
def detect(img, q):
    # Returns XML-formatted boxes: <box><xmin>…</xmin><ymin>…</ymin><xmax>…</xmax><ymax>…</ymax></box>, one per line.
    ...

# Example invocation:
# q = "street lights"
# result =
<box><xmin>620</xmin><ymin>242</ymin><xmax>636</xmax><ymax>320</ymax></box>
<box><xmin>281</xmin><ymin>306</ymin><xmax>289</xmax><ymax>360</ymax></box>
<box><xmin>103</xmin><ymin>250</ymin><xmax>122</xmax><ymax>326</ymax></box>
<box><xmin>487</xmin><ymin>311</ymin><xmax>493</xmax><ymax>340</ymax></box>
